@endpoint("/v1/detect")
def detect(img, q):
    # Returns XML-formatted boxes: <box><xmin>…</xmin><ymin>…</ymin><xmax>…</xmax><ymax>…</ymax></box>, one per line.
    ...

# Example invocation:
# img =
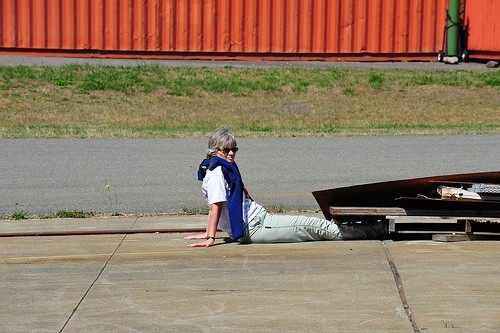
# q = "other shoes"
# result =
<box><xmin>366</xmin><ymin>221</ymin><xmax>387</xmax><ymax>240</ymax></box>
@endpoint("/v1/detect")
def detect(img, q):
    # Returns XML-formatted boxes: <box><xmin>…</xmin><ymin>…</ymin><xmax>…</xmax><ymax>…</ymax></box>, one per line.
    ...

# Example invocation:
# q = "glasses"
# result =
<box><xmin>219</xmin><ymin>147</ymin><xmax>239</xmax><ymax>154</ymax></box>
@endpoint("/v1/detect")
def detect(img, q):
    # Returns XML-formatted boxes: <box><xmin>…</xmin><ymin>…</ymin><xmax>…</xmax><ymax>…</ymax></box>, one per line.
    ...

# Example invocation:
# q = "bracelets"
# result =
<box><xmin>207</xmin><ymin>236</ymin><xmax>215</xmax><ymax>240</ymax></box>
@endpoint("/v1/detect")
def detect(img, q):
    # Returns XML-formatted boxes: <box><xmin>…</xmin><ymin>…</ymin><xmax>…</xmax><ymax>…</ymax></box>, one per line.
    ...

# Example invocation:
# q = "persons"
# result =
<box><xmin>184</xmin><ymin>127</ymin><xmax>391</xmax><ymax>248</ymax></box>
